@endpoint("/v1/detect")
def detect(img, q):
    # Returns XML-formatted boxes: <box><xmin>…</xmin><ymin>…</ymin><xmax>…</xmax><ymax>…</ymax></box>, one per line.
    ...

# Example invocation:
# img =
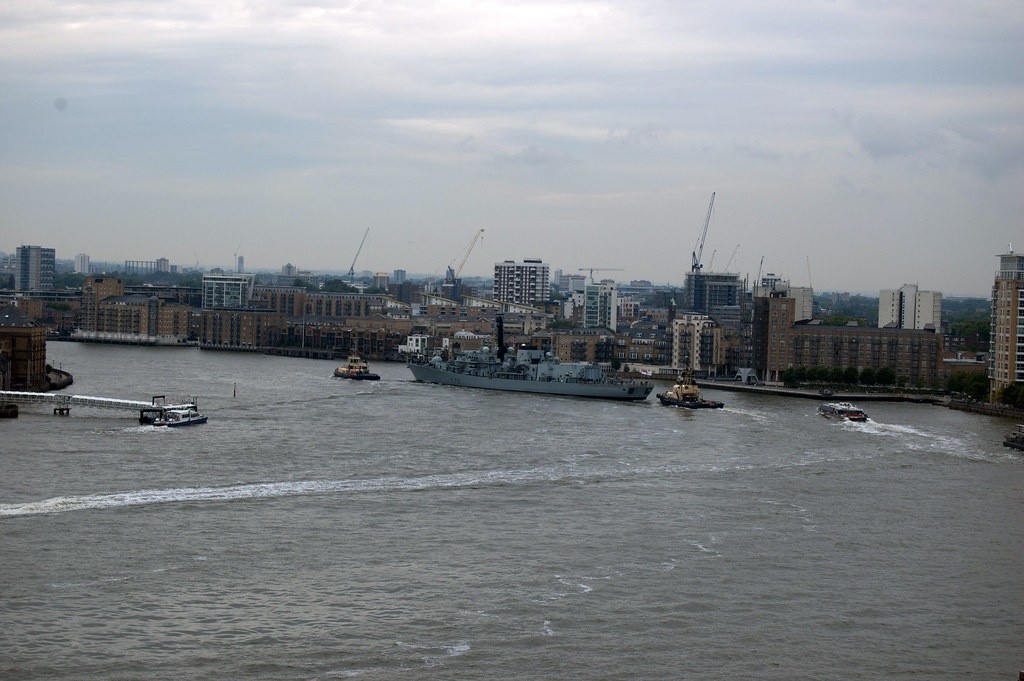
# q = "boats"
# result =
<box><xmin>154</xmin><ymin>409</ymin><xmax>209</xmax><ymax>428</ymax></box>
<box><xmin>818</xmin><ymin>401</ymin><xmax>871</xmax><ymax>424</ymax></box>
<box><xmin>658</xmin><ymin>366</ymin><xmax>728</xmax><ymax>410</ymax></box>
<box><xmin>1004</xmin><ymin>424</ymin><xmax>1024</xmax><ymax>450</ymax></box>
<box><xmin>333</xmin><ymin>354</ymin><xmax>381</xmax><ymax>382</ymax></box>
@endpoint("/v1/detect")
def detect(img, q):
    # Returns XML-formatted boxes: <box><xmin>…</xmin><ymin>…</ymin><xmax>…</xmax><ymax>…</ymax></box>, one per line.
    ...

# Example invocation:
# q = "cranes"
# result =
<box><xmin>578</xmin><ymin>267</ymin><xmax>623</xmax><ymax>279</ymax></box>
<box><xmin>689</xmin><ymin>191</ymin><xmax>717</xmax><ymax>274</ymax></box>
<box><xmin>447</xmin><ymin>229</ymin><xmax>485</xmax><ymax>282</ymax></box>
<box><xmin>347</xmin><ymin>227</ymin><xmax>371</xmax><ymax>284</ymax></box>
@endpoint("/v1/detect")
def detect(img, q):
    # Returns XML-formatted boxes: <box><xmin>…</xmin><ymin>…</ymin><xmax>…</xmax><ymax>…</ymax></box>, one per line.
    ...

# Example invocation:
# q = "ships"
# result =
<box><xmin>406</xmin><ymin>313</ymin><xmax>655</xmax><ymax>401</ymax></box>
